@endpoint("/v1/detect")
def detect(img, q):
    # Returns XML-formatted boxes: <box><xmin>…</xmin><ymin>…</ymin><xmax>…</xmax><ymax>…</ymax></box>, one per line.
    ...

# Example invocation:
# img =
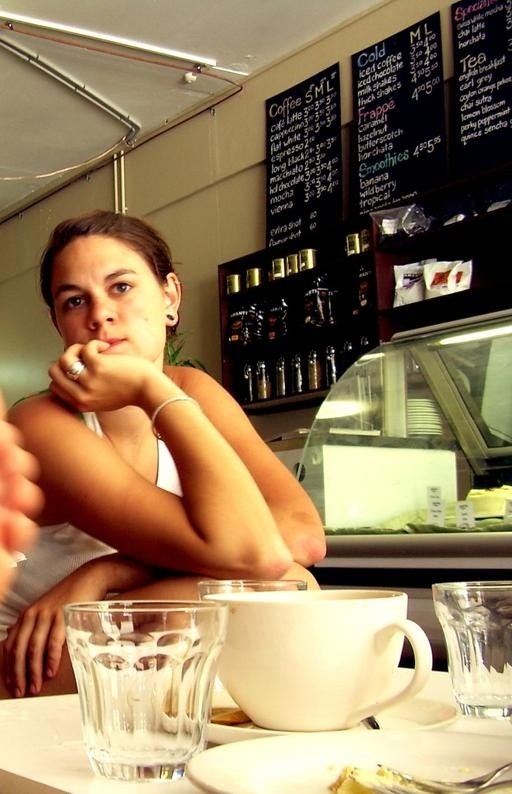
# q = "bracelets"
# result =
<box><xmin>150</xmin><ymin>395</ymin><xmax>198</xmax><ymax>441</ymax></box>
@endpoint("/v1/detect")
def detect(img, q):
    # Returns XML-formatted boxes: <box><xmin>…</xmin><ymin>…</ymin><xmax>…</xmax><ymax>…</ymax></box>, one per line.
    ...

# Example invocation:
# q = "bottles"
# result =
<box><xmin>246</xmin><ymin>268</ymin><xmax>263</xmax><ymax>288</ymax></box>
<box><xmin>241</xmin><ymin>363</ymin><xmax>256</xmax><ymax>404</ymax></box>
<box><xmin>323</xmin><ymin>345</ymin><xmax>338</xmax><ymax>390</ymax></box>
<box><xmin>257</xmin><ymin>360</ymin><xmax>272</xmax><ymax>401</ymax></box>
<box><xmin>357</xmin><ymin>336</ymin><xmax>370</xmax><ymax>361</ymax></box>
<box><xmin>292</xmin><ymin>354</ymin><xmax>307</xmax><ymax>395</ymax></box>
<box><xmin>275</xmin><ymin>357</ymin><xmax>291</xmax><ymax>399</ymax></box>
<box><xmin>361</xmin><ymin>228</ymin><xmax>371</xmax><ymax>253</ymax></box>
<box><xmin>299</xmin><ymin>248</ymin><xmax>317</xmax><ymax>272</ymax></box>
<box><xmin>285</xmin><ymin>254</ymin><xmax>299</xmax><ymax>277</ymax></box>
<box><xmin>226</xmin><ymin>274</ymin><xmax>243</xmax><ymax>295</ymax></box>
<box><xmin>270</xmin><ymin>257</ymin><xmax>285</xmax><ymax>281</ymax></box>
<box><xmin>308</xmin><ymin>349</ymin><xmax>323</xmax><ymax>391</ymax></box>
<box><xmin>345</xmin><ymin>232</ymin><xmax>361</xmax><ymax>256</ymax></box>
<box><xmin>340</xmin><ymin>341</ymin><xmax>355</xmax><ymax>374</ymax></box>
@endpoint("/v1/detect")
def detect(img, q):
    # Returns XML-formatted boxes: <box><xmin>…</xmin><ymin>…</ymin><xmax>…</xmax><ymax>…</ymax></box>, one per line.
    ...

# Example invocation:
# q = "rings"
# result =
<box><xmin>66</xmin><ymin>361</ymin><xmax>84</xmax><ymax>380</ymax></box>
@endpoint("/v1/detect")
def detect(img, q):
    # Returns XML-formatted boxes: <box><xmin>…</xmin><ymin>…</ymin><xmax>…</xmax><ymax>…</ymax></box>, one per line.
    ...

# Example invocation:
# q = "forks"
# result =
<box><xmin>377</xmin><ymin>762</ymin><xmax>512</xmax><ymax>792</ymax></box>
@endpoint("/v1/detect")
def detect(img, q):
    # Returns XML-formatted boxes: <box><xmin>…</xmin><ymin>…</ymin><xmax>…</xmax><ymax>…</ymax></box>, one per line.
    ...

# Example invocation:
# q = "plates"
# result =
<box><xmin>404</xmin><ymin>399</ymin><xmax>443</xmax><ymax>434</ymax></box>
<box><xmin>186</xmin><ymin>731</ymin><xmax>512</xmax><ymax>793</ymax></box>
<box><xmin>161</xmin><ymin>698</ymin><xmax>458</xmax><ymax>744</ymax></box>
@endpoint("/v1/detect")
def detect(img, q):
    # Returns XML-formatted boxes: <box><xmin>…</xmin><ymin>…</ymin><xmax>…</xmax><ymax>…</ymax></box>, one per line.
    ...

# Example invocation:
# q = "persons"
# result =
<box><xmin>1</xmin><ymin>393</ymin><xmax>46</xmax><ymax>600</ymax></box>
<box><xmin>0</xmin><ymin>211</ymin><xmax>327</xmax><ymax>701</ymax></box>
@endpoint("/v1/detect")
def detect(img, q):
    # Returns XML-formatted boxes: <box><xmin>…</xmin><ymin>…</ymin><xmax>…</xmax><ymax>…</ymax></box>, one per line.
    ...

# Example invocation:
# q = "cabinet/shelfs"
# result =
<box><xmin>294</xmin><ymin>309</ymin><xmax>512</xmax><ymax>571</ymax></box>
<box><xmin>216</xmin><ymin>184</ymin><xmax>512</xmax><ymax>415</ymax></box>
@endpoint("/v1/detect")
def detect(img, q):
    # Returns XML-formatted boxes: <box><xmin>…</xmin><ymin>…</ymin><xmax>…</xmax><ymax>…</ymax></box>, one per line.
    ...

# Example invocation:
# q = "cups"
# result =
<box><xmin>198</xmin><ymin>578</ymin><xmax>307</xmax><ymax>708</ymax></box>
<box><xmin>431</xmin><ymin>581</ymin><xmax>512</xmax><ymax>720</ymax></box>
<box><xmin>202</xmin><ymin>590</ymin><xmax>430</xmax><ymax>732</ymax></box>
<box><xmin>63</xmin><ymin>599</ymin><xmax>228</xmax><ymax>781</ymax></box>
